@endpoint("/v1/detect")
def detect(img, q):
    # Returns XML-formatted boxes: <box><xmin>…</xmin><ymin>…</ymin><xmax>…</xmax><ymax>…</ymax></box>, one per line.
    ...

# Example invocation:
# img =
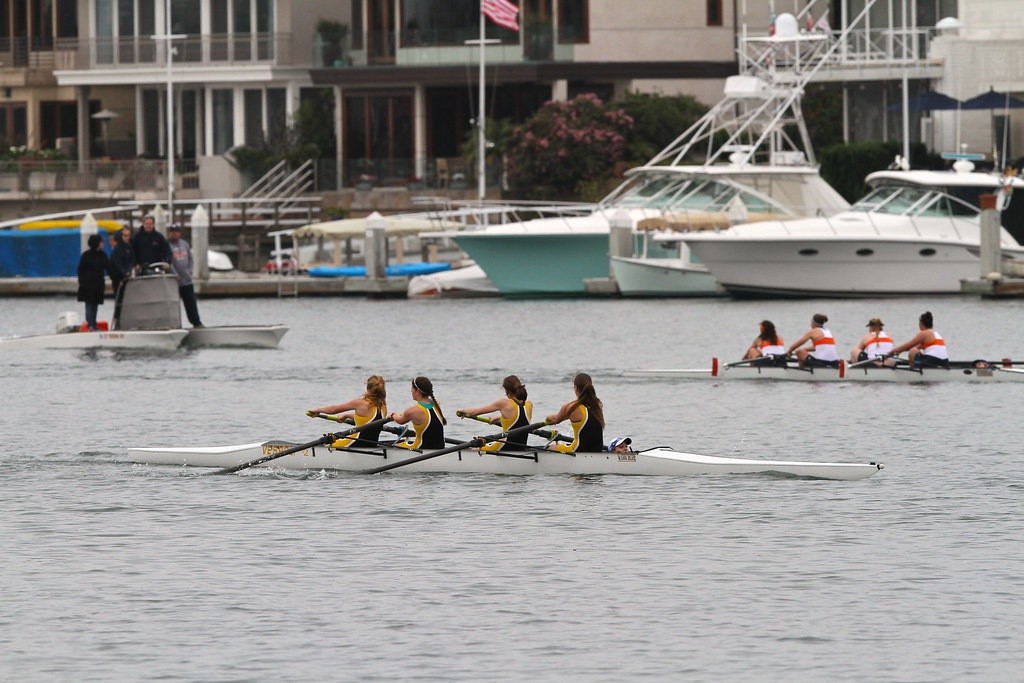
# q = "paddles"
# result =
<box><xmin>357</xmin><ymin>421</ymin><xmax>553</xmax><ymax>475</ymax></box>
<box><xmin>204</xmin><ymin>416</ymin><xmax>393</xmax><ymax>475</ymax></box>
<box><xmin>710</xmin><ymin>352</ymin><xmax>797</xmax><ymax>376</ymax></box>
<box><xmin>457</xmin><ymin>410</ymin><xmax>608</xmax><ymax>450</ymax></box>
<box><xmin>306</xmin><ymin>411</ymin><xmax>466</xmax><ymax>444</ymax></box>
<box><xmin>837</xmin><ymin>354</ymin><xmax>892</xmax><ymax>378</ymax></box>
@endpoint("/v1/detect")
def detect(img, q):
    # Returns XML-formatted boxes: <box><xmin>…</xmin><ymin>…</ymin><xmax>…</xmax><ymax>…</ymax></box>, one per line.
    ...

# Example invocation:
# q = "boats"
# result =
<box><xmin>607</xmin><ymin>212</ymin><xmax>789</xmax><ymax>299</ymax></box>
<box><xmin>0</xmin><ymin>204</ymin><xmax>594</xmax><ymax>301</ymax></box>
<box><xmin>125</xmin><ymin>439</ymin><xmax>886</xmax><ymax>481</ymax></box>
<box><xmin>619</xmin><ymin>364</ymin><xmax>1024</xmax><ymax>384</ymax></box>
<box><xmin>652</xmin><ymin>80</ymin><xmax>1024</xmax><ymax>301</ymax></box>
<box><xmin>0</xmin><ymin>261</ymin><xmax>290</xmax><ymax>351</ymax></box>
<box><xmin>423</xmin><ymin>1</ymin><xmax>873</xmax><ymax>301</ymax></box>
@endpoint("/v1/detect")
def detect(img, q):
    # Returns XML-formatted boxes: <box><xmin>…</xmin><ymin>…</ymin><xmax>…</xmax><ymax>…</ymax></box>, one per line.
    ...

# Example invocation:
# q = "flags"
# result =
<box><xmin>480</xmin><ymin>0</ymin><xmax>520</xmax><ymax>31</ymax></box>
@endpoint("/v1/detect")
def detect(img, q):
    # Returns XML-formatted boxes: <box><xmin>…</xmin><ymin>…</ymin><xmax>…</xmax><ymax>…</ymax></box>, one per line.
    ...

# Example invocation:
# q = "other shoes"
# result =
<box><xmin>88</xmin><ymin>328</ymin><xmax>96</xmax><ymax>332</ymax></box>
<box><xmin>195</xmin><ymin>324</ymin><xmax>206</xmax><ymax>328</ymax></box>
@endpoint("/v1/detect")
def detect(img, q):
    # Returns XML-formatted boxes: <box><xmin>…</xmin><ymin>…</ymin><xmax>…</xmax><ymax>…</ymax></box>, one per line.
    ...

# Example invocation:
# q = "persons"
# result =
<box><xmin>787</xmin><ymin>314</ymin><xmax>839</xmax><ymax>368</ymax></box>
<box><xmin>77</xmin><ymin>235</ymin><xmax>111</xmax><ymax>331</ymax></box>
<box><xmin>850</xmin><ymin>318</ymin><xmax>898</xmax><ymax>369</ymax></box>
<box><xmin>886</xmin><ymin>311</ymin><xmax>948</xmax><ymax>368</ymax></box>
<box><xmin>545</xmin><ymin>373</ymin><xmax>604</xmax><ymax>453</ymax></box>
<box><xmin>132</xmin><ymin>217</ymin><xmax>173</xmax><ymax>271</ymax></box>
<box><xmin>166</xmin><ymin>225</ymin><xmax>204</xmax><ymax>329</ymax></box>
<box><xmin>109</xmin><ymin>227</ymin><xmax>143</xmax><ymax>299</ymax></box>
<box><xmin>460</xmin><ymin>375</ymin><xmax>532</xmax><ymax>451</ymax></box>
<box><xmin>309</xmin><ymin>377</ymin><xmax>387</xmax><ymax>448</ymax></box>
<box><xmin>608</xmin><ymin>437</ymin><xmax>632</xmax><ymax>453</ymax></box>
<box><xmin>387</xmin><ymin>376</ymin><xmax>447</xmax><ymax>449</ymax></box>
<box><xmin>742</xmin><ymin>321</ymin><xmax>785</xmax><ymax>360</ymax></box>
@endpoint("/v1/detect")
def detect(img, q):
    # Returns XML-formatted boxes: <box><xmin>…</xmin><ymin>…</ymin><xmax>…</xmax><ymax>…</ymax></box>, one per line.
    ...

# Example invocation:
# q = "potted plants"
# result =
<box><xmin>315</xmin><ymin>18</ymin><xmax>348</xmax><ymax>67</ymax></box>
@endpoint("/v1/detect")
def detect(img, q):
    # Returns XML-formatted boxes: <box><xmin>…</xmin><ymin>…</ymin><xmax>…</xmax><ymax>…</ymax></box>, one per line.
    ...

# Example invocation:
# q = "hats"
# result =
<box><xmin>607</xmin><ymin>438</ymin><xmax>632</xmax><ymax>451</ymax></box>
<box><xmin>88</xmin><ymin>234</ymin><xmax>101</xmax><ymax>251</ymax></box>
<box><xmin>864</xmin><ymin>319</ymin><xmax>885</xmax><ymax>327</ymax></box>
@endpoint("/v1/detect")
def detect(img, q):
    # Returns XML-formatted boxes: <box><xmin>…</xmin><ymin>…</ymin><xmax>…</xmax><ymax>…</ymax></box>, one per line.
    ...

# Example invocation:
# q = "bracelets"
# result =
<box><xmin>391</xmin><ymin>413</ymin><xmax>394</xmax><ymax>417</ymax></box>
